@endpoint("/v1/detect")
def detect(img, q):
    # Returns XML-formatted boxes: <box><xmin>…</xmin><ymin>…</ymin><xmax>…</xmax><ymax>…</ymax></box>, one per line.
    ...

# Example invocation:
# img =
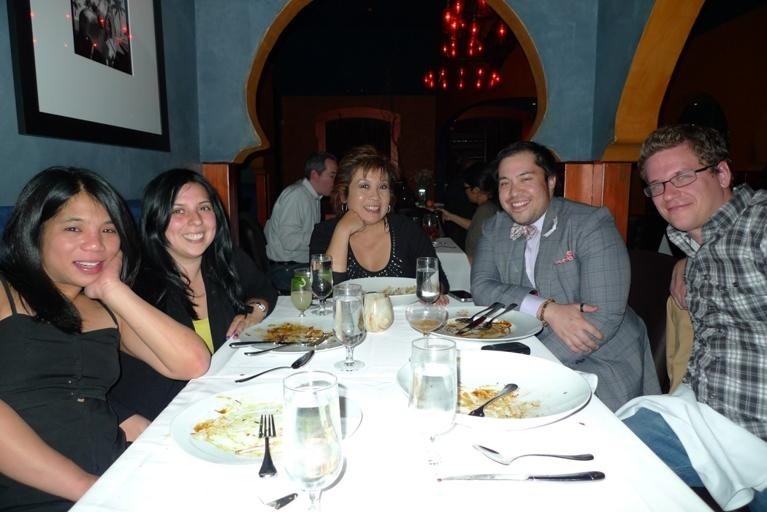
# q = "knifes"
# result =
<box><xmin>436</xmin><ymin>470</ymin><xmax>607</xmax><ymax>483</ymax></box>
<box><xmin>457</xmin><ymin>304</ymin><xmax>504</xmax><ymax>334</ymax></box>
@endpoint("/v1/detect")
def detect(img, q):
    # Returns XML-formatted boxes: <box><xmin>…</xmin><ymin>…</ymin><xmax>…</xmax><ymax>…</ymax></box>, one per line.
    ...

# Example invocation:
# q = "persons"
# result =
<box><xmin>258</xmin><ymin>150</ymin><xmax>339</xmax><ymax>293</ymax></box>
<box><xmin>0</xmin><ymin>165</ymin><xmax>210</xmax><ymax>510</ymax></box>
<box><xmin>304</xmin><ymin>143</ymin><xmax>422</xmax><ymax>295</ymax></box>
<box><xmin>107</xmin><ymin>166</ymin><xmax>278</xmax><ymax>444</ymax></box>
<box><xmin>600</xmin><ymin>120</ymin><xmax>765</xmax><ymax>510</ymax></box>
<box><xmin>469</xmin><ymin>139</ymin><xmax>666</xmax><ymax>414</ymax></box>
<box><xmin>426</xmin><ymin>165</ymin><xmax>500</xmax><ymax>263</ymax></box>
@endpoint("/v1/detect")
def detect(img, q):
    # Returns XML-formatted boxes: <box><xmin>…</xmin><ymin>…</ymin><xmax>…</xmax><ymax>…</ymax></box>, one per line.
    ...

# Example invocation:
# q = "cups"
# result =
<box><xmin>417</xmin><ymin>186</ymin><xmax>427</xmax><ymax>208</ymax></box>
<box><xmin>359</xmin><ymin>292</ymin><xmax>394</xmax><ymax>331</ymax></box>
<box><xmin>421</xmin><ymin>214</ymin><xmax>441</xmax><ymax>240</ymax></box>
<box><xmin>415</xmin><ymin>257</ymin><xmax>439</xmax><ymax>306</ymax></box>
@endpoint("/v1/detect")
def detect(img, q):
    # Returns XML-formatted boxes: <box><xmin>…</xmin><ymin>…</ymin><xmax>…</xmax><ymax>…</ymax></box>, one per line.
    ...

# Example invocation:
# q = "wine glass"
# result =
<box><xmin>331</xmin><ymin>283</ymin><xmax>366</xmax><ymax>370</ymax></box>
<box><xmin>288</xmin><ymin>254</ymin><xmax>333</xmax><ymax>319</ymax></box>
<box><xmin>280</xmin><ymin>370</ymin><xmax>345</xmax><ymax>510</ymax></box>
<box><xmin>404</xmin><ymin>338</ymin><xmax>459</xmax><ymax>468</ymax></box>
<box><xmin>404</xmin><ymin>304</ymin><xmax>448</xmax><ymax>348</ymax></box>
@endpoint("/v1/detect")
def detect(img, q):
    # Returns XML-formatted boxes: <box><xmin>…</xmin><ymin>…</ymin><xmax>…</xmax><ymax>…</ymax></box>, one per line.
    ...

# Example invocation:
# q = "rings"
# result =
<box><xmin>578</xmin><ymin>301</ymin><xmax>587</xmax><ymax>314</ymax></box>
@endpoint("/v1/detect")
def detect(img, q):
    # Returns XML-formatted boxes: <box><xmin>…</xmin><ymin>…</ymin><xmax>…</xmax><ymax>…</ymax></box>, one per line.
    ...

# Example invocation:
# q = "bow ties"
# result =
<box><xmin>509</xmin><ymin>222</ymin><xmax>536</xmax><ymax>241</ymax></box>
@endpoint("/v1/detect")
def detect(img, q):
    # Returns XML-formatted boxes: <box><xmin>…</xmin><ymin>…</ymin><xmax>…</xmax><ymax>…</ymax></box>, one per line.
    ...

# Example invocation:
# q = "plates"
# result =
<box><xmin>395</xmin><ymin>351</ymin><xmax>591</xmax><ymax>428</ymax></box>
<box><xmin>170</xmin><ymin>381</ymin><xmax>364</xmax><ymax>463</ymax></box>
<box><xmin>237</xmin><ymin>315</ymin><xmax>363</xmax><ymax>352</ymax></box>
<box><xmin>432</xmin><ymin>306</ymin><xmax>543</xmax><ymax>343</ymax></box>
<box><xmin>336</xmin><ymin>276</ymin><xmax>419</xmax><ymax>305</ymax></box>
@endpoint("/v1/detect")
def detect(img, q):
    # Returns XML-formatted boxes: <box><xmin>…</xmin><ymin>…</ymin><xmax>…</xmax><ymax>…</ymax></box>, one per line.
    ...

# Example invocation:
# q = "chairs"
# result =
<box><xmin>242</xmin><ymin>223</ymin><xmax>270</xmax><ymax>269</ymax></box>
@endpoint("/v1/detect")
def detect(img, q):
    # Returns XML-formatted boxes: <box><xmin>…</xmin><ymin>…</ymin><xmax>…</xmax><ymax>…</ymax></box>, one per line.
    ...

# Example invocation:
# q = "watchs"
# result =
<box><xmin>246</xmin><ymin>298</ymin><xmax>267</xmax><ymax>319</ymax></box>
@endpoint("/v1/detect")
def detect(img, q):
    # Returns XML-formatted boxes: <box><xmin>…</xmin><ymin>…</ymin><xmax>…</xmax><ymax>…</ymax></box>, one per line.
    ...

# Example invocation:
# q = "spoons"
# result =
<box><xmin>479</xmin><ymin>302</ymin><xmax>516</xmax><ymax>330</ymax></box>
<box><xmin>235</xmin><ymin>349</ymin><xmax>316</xmax><ymax>384</ymax></box>
<box><xmin>455</xmin><ymin>301</ymin><xmax>500</xmax><ymax>324</ymax></box>
<box><xmin>472</xmin><ymin>442</ymin><xmax>593</xmax><ymax>464</ymax></box>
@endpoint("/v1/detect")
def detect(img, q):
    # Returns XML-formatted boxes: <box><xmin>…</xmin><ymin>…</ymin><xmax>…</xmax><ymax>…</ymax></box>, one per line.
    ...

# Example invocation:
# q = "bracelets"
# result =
<box><xmin>538</xmin><ymin>297</ymin><xmax>556</xmax><ymax>329</ymax></box>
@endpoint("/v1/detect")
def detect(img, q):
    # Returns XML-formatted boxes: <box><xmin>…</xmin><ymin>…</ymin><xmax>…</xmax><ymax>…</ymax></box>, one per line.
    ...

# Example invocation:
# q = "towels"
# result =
<box><xmin>599</xmin><ymin>382</ymin><xmax>767</xmax><ymax>510</ymax></box>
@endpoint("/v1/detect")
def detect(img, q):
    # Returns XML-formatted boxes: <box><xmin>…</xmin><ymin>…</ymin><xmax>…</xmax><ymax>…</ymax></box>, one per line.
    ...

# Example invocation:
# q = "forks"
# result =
<box><xmin>468</xmin><ymin>380</ymin><xmax>519</xmax><ymax>418</ymax></box>
<box><xmin>229</xmin><ymin>337</ymin><xmax>327</xmax><ymax>359</ymax></box>
<box><xmin>258</xmin><ymin>414</ymin><xmax>277</xmax><ymax>478</ymax></box>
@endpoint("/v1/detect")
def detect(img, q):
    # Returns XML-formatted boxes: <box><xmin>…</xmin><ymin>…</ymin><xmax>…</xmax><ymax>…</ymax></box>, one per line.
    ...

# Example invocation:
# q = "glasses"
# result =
<box><xmin>643</xmin><ymin>165</ymin><xmax>713</xmax><ymax>197</ymax></box>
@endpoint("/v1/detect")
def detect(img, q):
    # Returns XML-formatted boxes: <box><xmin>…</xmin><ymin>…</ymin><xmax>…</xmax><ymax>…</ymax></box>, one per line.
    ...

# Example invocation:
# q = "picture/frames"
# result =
<box><xmin>7</xmin><ymin>1</ymin><xmax>173</xmax><ymax>158</ymax></box>
